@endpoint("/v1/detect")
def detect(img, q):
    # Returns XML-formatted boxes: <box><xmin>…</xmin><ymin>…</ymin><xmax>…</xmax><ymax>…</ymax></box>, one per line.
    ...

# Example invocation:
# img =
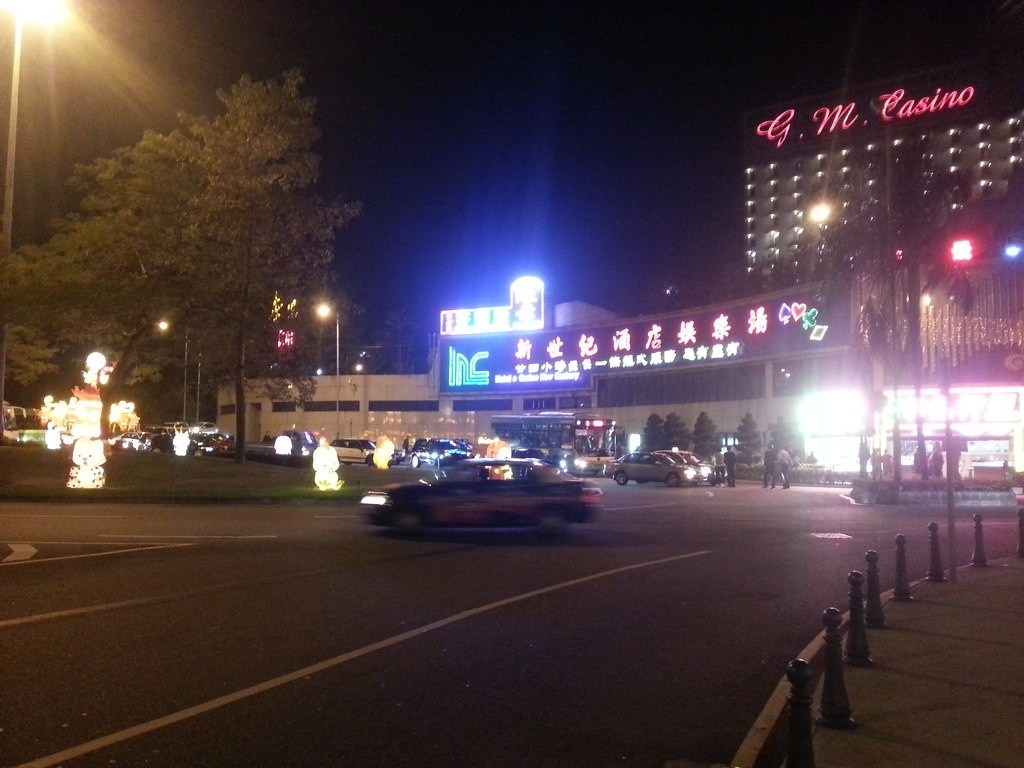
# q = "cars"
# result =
<box><xmin>328</xmin><ymin>436</ymin><xmax>388</xmax><ymax>466</ymax></box>
<box><xmin>360</xmin><ymin>458</ymin><xmax>603</xmax><ymax>539</ymax></box>
<box><xmin>663</xmin><ymin>451</ymin><xmax>708</xmax><ymax>470</ymax></box>
<box><xmin>71</xmin><ymin>420</ymin><xmax>236</xmax><ymax>459</ymax></box>
<box><xmin>410</xmin><ymin>438</ymin><xmax>470</xmax><ymax>468</ymax></box>
<box><xmin>616</xmin><ymin>454</ymin><xmax>698</xmax><ymax>486</ymax></box>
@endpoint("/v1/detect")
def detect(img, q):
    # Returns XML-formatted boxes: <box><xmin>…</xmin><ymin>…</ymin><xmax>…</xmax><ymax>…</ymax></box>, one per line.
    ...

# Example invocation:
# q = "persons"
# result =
<box><xmin>859</xmin><ymin>443</ymin><xmax>944</xmax><ymax>479</ymax></box>
<box><xmin>403</xmin><ymin>436</ymin><xmax>409</xmax><ymax>452</ymax></box>
<box><xmin>777</xmin><ymin>446</ymin><xmax>793</xmax><ymax>489</ymax></box>
<box><xmin>764</xmin><ymin>443</ymin><xmax>778</xmax><ymax>490</ymax></box>
<box><xmin>723</xmin><ymin>446</ymin><xmax>736</xmax><ymax>487</ymax></box>
<box><xmin>715</xmin><ymin>448</ymin><xmax>724</xmax><ymax>486</ymax></box>
<box><xmin>806</xmin><ymin>452</ymin><xmax>817</xmax><ymax>464</ymax></box>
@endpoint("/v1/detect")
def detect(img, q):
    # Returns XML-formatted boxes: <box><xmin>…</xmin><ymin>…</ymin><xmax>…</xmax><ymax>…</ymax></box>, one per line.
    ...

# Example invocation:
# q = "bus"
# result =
<box><xmin>1</xmin><ymin>399</ymin><xmax>26</xmax><ymax>445</ymax></box>
<box><xmin>486</xmin><ymin>411</ymin><xmax>612</xmax><ymax>477</ymax></box>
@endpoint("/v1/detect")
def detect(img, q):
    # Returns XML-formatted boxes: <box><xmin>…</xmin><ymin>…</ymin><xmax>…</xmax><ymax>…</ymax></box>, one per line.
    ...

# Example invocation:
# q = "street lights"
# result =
<box><xmin>158</xmin><ymin>318</ymin><xmax>188</xmax><ymax>421</ymax></box>
<box><xmin>315</xmin><ymin>303</ymin><xmax>341</xmax><ymax>457</ymax></box>
<box><xmin>809</xmin><ymin>205</ymin><xmax>834</xmax><ymax>302</ymax></box>
<box><xmin>0</xmin><ymin>2</ymin><xmax>42</xmax><ymax>324</ymax></box>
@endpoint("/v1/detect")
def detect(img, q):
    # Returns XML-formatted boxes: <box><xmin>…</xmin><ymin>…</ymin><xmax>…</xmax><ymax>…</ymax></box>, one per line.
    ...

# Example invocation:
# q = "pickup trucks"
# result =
<box><xmin>245</xmin><ymin>429</ymin><xmax>319</xmax><ymax>467</ymax></box>
